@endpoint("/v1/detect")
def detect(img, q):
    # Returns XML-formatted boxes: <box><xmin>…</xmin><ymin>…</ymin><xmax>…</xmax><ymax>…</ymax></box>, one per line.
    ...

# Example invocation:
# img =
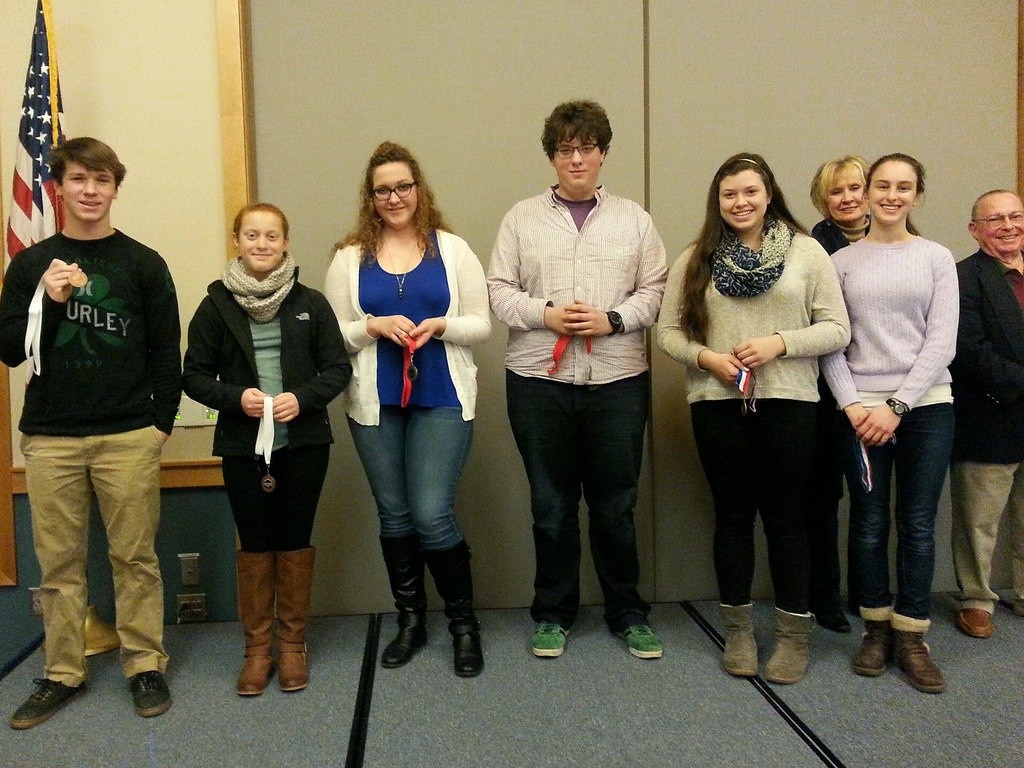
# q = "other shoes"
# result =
<box><xmin>531</xmin><ymin>620</ymin><xmax>570</xmax><ymax>657</ymax></box>
<box><xmin>959</xmin><ymin>609</ymin><xmax>993</xmax><ymax>638</ymax></box>
<box><xmin>616</xmin><ymin>624</ymin><xmax>663</xmax><ymax>657</ymax></box>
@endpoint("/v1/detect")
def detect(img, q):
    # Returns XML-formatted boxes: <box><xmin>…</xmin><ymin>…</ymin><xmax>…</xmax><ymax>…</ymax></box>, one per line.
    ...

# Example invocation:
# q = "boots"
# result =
<box><xmin>719</xmin><ymin>601</ymin><xmax>758</xmax><ymax>675</ymax></box>
<box><xmin>765</xmin><ymin>605</ymin><xmax>816</xmax><ymax>683</ymax></box>
<box><xmin>424</xmin><ymin>537</ymin><xmax>485</xmax><ymax>677</ymax></box>
<box><xmin>888</xmin><ymin>610</ymin><xmax>947</xmax><ymax>693</ymax></box>
<box><xmin>235</xmin><ymin>550</ymin><xmax>277</xmax><ymax>695</ymax></box>
<box><xmin>379</xmin><ymin>532</ymin><xmax>428</xmax><ymax>669</ymax></box>
<box><xmin>275</xmin><ymin>547</ymin><xmax>316</xmax><ymax>691</ymax></box>
<box><xmin>854</xmin><ymin>605</ymin><xmax>893</xmax><ymax>675</ymax></box>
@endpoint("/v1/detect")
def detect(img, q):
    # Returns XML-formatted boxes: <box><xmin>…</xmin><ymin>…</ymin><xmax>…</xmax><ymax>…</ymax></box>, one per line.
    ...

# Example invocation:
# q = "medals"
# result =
<box><xmin>741</xmin><ymin>401</ymin><xmax>747</xmax><ymax>416</ymax></box>
<box><xmin>408</xmin><ymin>365</ymin><xmax>417</xmax><ymax>381</ymax></box>
<box><xmin>69</xmin><ymin>269</ymin><xmax>88</xmax><ymax>287</ymax></box>
<box><xmin>261</xmin><ymin>475</ymin><xmax>275</xmax><ymax>494</ymax></box>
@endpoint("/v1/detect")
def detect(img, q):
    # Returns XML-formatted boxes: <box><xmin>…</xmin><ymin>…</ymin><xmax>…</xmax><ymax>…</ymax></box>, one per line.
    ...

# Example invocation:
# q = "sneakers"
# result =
<box><xmin>128</xmin><ymin>670</ymin><xmax>172</xmax><ymax>717</ymax></box>
<box><xmin>10</xmin><ymin>678</ymin><xmax>88</xmax><ymax>730</ymax></box>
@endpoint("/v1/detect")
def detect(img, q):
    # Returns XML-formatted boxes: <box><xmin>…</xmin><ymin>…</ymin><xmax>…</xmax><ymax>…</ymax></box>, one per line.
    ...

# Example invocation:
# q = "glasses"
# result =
<box><xmin>970</xmin><ymin>211</ymin><xmax>1024</xmax><ymax>228</ymax></box>
<box><xmin>371</xmin><ymin>181</ymin><xmax>416</xmax><ymax>202</ymax></box>
<box><xmin>553</xmin><ymin>143</ymin><xmax>598</xmax><ymax>158</ymax></box>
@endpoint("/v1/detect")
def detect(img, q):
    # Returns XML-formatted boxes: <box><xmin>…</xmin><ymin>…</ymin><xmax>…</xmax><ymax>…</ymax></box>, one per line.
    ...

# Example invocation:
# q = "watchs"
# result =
<box><xmin>606</xmin><ymin>311</ymin><xmax>622</xmax><ymax>337</ymax></box>
<box><xmin>886</xmin><ymin>398</ymin><xmax>907</xmax><ymax>418</ymax></box>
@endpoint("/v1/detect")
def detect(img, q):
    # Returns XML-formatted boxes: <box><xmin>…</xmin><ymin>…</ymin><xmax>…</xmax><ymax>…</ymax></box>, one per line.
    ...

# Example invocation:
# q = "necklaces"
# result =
<box><xmin>384</xmin><ymin>240</ymin><xmax>412</xmax><ymax>298</ymax></box>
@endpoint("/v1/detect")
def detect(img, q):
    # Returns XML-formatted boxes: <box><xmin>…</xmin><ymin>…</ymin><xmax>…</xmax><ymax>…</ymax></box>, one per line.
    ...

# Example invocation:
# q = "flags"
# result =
<box><xmin>5</xmin><ymin>0</ymin><xmax>65</xmax><ymax>279</ymax></box>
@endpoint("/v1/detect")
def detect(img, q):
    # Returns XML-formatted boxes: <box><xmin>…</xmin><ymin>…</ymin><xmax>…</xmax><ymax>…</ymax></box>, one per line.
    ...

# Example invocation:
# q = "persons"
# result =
<box><xmin>656</xmin><ymin>151</ymin><xmax>851</xmax><ymax>682</ymax></box>
<box><xmin>182</xmin><ymin>204</ymin><xmax>353</xmax><ymax>696</ymax></box>
<box><xmin>948</xmin><ymin>190</ymin><xmax>1024</xmax><ymax>639</ymax></box>
<box><xmin>809</xmin><ymin>157</ymin><xmax>871</xmax><ymax>633</ymax></box>
<box><xmin>1</xmin><ymin>137</ymin><xmax>183</xmax><ymax>729</ymax></box>
<box><xmin>821</xmin><ymin>152</ymin><xmax>960</xmax><ymax>693</ymax></box>
<box><xmin>487</xmin><ymin>98</ymin><xmax>668</xmax><ymax>661</ymax></box>
<box><xmin>322</xmin><ymin>142</ymin><xmax>492</xmax><ymax>676</ymax></box>
